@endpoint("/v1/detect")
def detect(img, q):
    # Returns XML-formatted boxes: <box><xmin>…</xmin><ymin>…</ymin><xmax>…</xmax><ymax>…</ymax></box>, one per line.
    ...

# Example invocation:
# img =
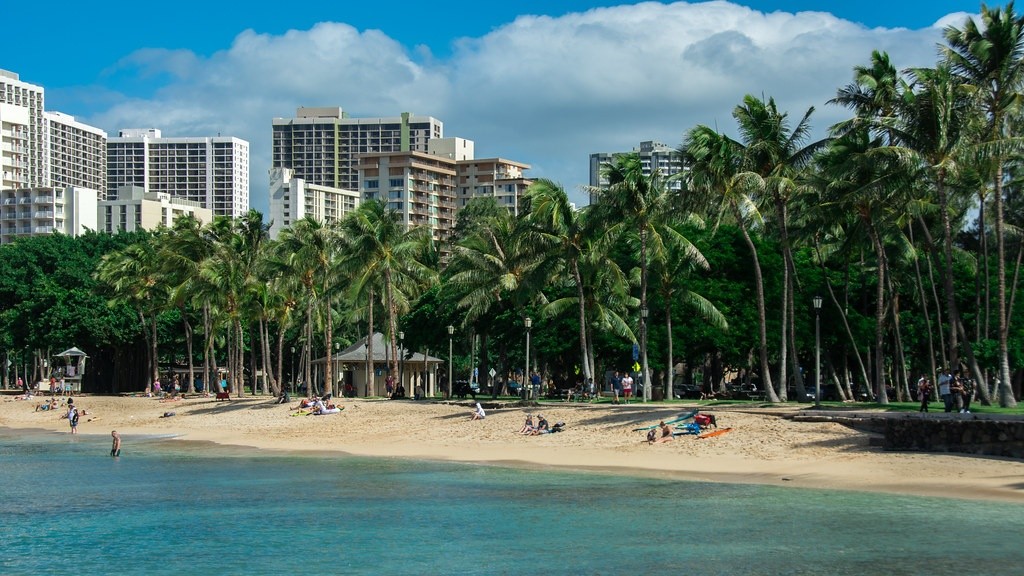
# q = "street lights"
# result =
<box><xmin>334</xmin><ymin>342</ymin><xmax>340</xmax><ymax>398</ymax></box>
<box><xmin>523</xmin><ymin>317</ymin><xmax>532</xmax><ymax>402</ymax></box>
<box><xmin>397</xmin><ymin>330</ymin><xmax>405</xmax><ymax>398</ymax></box>
<box><xmin>363</xmin><ymin>337</ymin><xmax>369</xmax><ymax>398</ymax></box>
<box><xmin>290</xmin><ymin>346</ymin><xmax>295</xmax><ymax>395</ymax></box>
<box><xmin>640</xmin><ymin>303</ymin><xmax>650</xmax><ymax>405</ymax></box>
<box><xmin>811</xmin><ymin>294</ymin><xmax>823</xmax><ymax>407</ymax></box>
<box><xmin>446</xmin><ymin>324</ymin><xmax>454</xmax><ymax>401</ymax></box>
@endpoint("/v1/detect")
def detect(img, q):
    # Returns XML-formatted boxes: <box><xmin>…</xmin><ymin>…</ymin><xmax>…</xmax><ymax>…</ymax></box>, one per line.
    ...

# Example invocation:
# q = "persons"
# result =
<box><xmin>647</xmin><ymin>421</ymin><xmax>674</xmax><ymax>446</ymax></box>
<box><xmin>519</xmin><ymin>413</ymin><xmax>534</xmax><ymax>433</ymax></box>
<box><xmin>321</xmin><ymin>376</ymin><xmax>325</xmax><ymax>395</ymax></box>
<box><xmin>414</xmin><ymin>386</ymin><xmax>424</xmax><ymax>400</ymax></box>
<box><xmin>144</xmin><ymin>384</ymin><xmax>154</xmax><ymax>397</ymax></box>
<box><xmin>36</xmin><ymin>397</ymin><xmax>58</xmax><ymax>412</ymax></box>
<box><xmin>283</xmin><ymin>378</ymin><xmax>307</xmax><ymax>394</ymax></box>
<box><xmin>290</xmin><ymin>393</ymin><xmax>345</xmax><ymax>415</ymax></box>
<box><xmin>49</xmin><ymin>375</ymin><xmax>56</xmax><ymax>396</ymax></box>
<box><xmin>153</xmin><ymin>378</ymin><xmax>162</xmax><ymax>395</ymax></box>
<box><xmin>567</xmin><ymin>377</ymin><xmax>594</xmax><ymax>403</ymax></box>
<box><xmin>383</xmin><ymin>373</ymin><xmax>404</xmax><ymax>397</ymax></box>
<box><xmin>60</xmin><ymin>376</ymin><xmax>74</xmax><ymax>396</ymax></box>
<box><xmin>338</xmin><ymin>378</ymin><xmax>354</xmax><ymax>398</ymax></box>
<box><xmin>700</xmin><ymin>389</ymin><xmax>716</xmax><ymax>401</ymax></box>
<box><xmin>528</xmin><ymin>414</ymin><xmax>549</xmax><ymax>435</ymax></box>
<box><xmin>68</xmin><ymin>405</ymin><xmax>79</xmax><ymax>434</ymax></box>
<box><xmin>164</xmin><ymin>375</ymin><xmax>203</xmax><ymax>392</ymax></box>
<box><xmin>18</xmin><ymin>377</ymin><xmax>22</xmax><ymax>386</ymax></box>
<box><xmin>110</xmin><ymin>430</ymin><xmax>121</xmax><ymax>457</ymax></box>
<box><xmin>917</xmin><ymin>369</ymin><xmax>973</xmax><ymax>413</ymax></box>
<box><xmin>440</xmin><ymin>373</ymin><xmax>447</xmax><ymax>397</ymax></box>
<box><xmin>274</xmin><ymin>390</ymin><xmax>286</xmax><ymax>403</ymax></box>
<box><xmin>610</xmin><ymin>370</ymin><xmax>633</xmax><ymax>404</ymax></box>
<box><xmin>469</xmin><ymin>402</ymin><xmax>485</xmax><ymax>420</ymax></box>
